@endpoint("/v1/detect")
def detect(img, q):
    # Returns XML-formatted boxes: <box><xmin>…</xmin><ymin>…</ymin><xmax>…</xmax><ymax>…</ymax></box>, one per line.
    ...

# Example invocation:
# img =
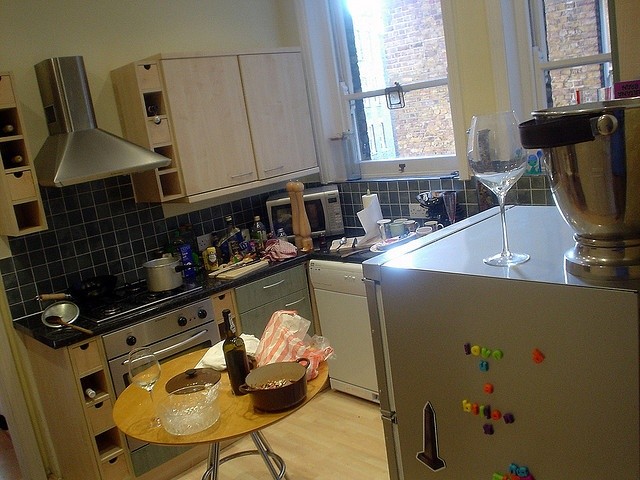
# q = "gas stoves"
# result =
<box><xmin>75</xmin><ymin>272</ymin><xmax>206</xmax><ymax>325</ymax></box>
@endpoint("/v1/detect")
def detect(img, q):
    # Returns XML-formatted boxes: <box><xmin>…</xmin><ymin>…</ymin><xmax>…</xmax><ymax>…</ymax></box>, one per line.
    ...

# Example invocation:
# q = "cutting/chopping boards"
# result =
<box><xmin>208</xmin><ymin>258</ymin><xmax>269</xmax><ymax>281</ymax></box>
<box><xmin>329</xmin><ymin>236</ymin><xmax>373</xmax><ymax>254</ymax></box>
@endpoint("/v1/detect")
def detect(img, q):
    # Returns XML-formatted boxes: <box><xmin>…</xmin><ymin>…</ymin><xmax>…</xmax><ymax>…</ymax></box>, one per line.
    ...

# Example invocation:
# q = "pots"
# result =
<box><xmin>143</xmin><ymin>258</ymin><xmax>196</xmax><ymax>293</ymax></box>
<box><xmin>35</xmin><ymin>277</ymin><xmax>120</xmax><ymax>307</ymax></box>
<box><xmin>238</xmin><ymin>358</ymin><xmax>309</xmax><ymax>413</ymax></box>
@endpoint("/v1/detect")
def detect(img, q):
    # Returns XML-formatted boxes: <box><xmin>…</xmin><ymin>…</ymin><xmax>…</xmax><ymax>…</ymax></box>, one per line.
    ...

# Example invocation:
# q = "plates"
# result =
<box><xmin>370</xmin><ymin>242</ymin><xmax>385</xmax><ymax>253</ymax></box>
<box><xmin>377</xmin><ymin>233</ymin><xmax>417</xmax><ymax>250</ymax></box>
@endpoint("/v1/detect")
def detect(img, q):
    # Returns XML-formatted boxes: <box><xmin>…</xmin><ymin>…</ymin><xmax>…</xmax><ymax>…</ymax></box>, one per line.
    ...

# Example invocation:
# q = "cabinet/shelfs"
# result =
<box><xmin>0</xmin><ymin>68</ymin><xmax>50</xmax><ymax>239</ymax></box>
<box><xmin>110</xmin><ymin>46</ymin><xmax>321</xmax><ymax>205</ymax></box>
<box><xmin>19</xmin><ymin>337</ymin><xmax>132</xmax><ymax>476</ymax></box>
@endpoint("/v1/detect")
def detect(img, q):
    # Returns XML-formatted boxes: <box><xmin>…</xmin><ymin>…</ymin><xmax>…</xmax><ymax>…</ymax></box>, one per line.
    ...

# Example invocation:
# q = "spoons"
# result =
<box><xmin>337</xmin><ymin>237</ymin><xmax>346</xmax><ymax>250</ymax></box>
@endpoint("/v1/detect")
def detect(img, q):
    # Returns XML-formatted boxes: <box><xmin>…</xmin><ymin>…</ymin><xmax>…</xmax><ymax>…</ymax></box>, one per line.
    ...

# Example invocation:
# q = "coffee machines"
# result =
<box><xmin>415</xmin><ymin>190</ymin><xmax>456</xmax><ymax>227</ymax></box>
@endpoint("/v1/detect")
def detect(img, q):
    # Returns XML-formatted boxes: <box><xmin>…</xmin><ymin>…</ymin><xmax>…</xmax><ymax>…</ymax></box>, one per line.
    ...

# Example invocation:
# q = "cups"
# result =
<box><xmin>425</xmin><ymin>220</ymin><xmax>444</xmax><ymax>232</ymax></box>
<box><xmin>416</xmin><ymin>226</ymin><xmax>432</xmax><ymax>236</ymax></box>
<box><xmin>404</xmin><ymin>220</ymin><xmax>419</xmax><ymax>233</ymax></box>
<box><xmin>390</xmin><ymin>223</ymin><xmax>409</xmax><ymax>238</ymax></box>
<box><xmin>394</xmin><ymin>217</ymin><xmax>408</xmax><ymax>222</ymax></box>
<box><xmin>377</xmin><ymin>219</ymin><xmax>391</xmax><ymax>239</ymax></box>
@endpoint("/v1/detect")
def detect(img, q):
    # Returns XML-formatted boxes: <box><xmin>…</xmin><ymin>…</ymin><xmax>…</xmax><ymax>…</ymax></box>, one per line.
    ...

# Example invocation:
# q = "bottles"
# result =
<box><xmin>253</xmin><ymin>215</ymin><xmax>265</xmax><ymax>239</ymax></box>
<box><xmin>329</xmin><ymin>133</ymin><xmax>347</xmax><ymax>181</ymax></box>
<box><xmin>179</xmin><ymin>226</ymin><xmax>188</xmax><ymax>243</ymax></box>
<box><xmin>172</xmin><ymin>231</ymin><xmax>183</xmax><ymax>249</ymax></box>
<box><xmin>3</xmin><ymin>120</ymin><xmax>15</xmax><ymax>131</ymax></box>
<box><xmin>184</xmin><ymin>224</ymin><xmax>195</xmax><ymax>244</ymax></box>
<box><xmin>342</xmin><ymin>129</ymin><xmax>361</xmax><ymax>181</ymax></box>
<box><xmin>10</xmin><ymin>153</ymin><xmax>24</xmax><ymax>164</ymax></box>
<box><xmin>148</xmin><ymin>103</ymin><xmax>159</xmax><ymax>113</ymax></box>
<box><xmin>86</xmin><ymin>385</ymin><xmax>95</xmax><ymax>398</ymax></box>
<box><xmin>222</xmin><ymin>309</ymin><xmax>250</xmax><ymax>395</ymax></box>
<box><xmin>226</xmin><ymin>216</ymin><xmax>233</xmax><ymax>236</ymax></box>
<box><xmin>277</xmin><ymin>228</ymin><xmax>286</xmax><ymax>244</ymax></box>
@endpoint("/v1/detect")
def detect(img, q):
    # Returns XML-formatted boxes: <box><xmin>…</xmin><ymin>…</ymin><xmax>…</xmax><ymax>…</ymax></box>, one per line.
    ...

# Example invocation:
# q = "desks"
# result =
<box><xmin>111</xmin><ymin>345</ymin><xmax>330</xmax><ymax>480</ymax></box>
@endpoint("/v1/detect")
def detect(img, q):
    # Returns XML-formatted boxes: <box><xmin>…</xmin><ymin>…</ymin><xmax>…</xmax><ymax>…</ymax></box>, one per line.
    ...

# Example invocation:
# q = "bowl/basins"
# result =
<box><xmin>156</xmin><ymin>385</ymin><xmax>220</xmax><ymax>435</ymax></box>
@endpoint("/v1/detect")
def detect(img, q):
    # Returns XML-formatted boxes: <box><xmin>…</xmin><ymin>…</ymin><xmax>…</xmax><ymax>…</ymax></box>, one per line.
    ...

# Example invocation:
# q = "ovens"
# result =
<box><xmin>101</xmin><ymin>297</ymin><xmax>251</xmax><ymax>452</ymax></box>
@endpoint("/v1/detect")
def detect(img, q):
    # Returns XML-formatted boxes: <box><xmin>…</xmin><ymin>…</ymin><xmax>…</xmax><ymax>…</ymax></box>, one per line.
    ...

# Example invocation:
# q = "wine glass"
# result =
<box><xmin>469</xmin><ymin>113</ymin><xmax>527</xmax><ymax>267</ymax></box>
<box><xmin>128</xmin><ymin>348</ymin><xmax>164</xmax><ymax>426</ymax></box>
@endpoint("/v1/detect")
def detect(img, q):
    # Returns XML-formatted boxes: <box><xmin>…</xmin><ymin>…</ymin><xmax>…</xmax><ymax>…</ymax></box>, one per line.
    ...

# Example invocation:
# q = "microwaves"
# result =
<box><xmin>266</xmin><ymin>185</ymin><xmax>345</xmax><ymax>242</ymax></box>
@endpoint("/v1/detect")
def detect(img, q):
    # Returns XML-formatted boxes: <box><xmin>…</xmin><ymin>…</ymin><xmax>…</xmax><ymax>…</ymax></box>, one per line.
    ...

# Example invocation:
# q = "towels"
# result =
<box><xmin>200</xmin><ymin>332</ymin><xmax>260</xmax><ymax>373</ymax></box>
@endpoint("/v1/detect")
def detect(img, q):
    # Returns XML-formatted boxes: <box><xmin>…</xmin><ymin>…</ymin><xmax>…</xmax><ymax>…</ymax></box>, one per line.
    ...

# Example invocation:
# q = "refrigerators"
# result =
<box><xmin>362</xmin><ymin>205</ymin><xmax>640</xmax><ymax>480</ymax></box>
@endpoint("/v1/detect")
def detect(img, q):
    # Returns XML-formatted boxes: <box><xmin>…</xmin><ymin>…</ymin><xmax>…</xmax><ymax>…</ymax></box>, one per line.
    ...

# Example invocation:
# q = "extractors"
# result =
<box><xmin>33</xmin><ymin>56</ymin><xmax>172</xmax><ymax>189</ymax></box>
